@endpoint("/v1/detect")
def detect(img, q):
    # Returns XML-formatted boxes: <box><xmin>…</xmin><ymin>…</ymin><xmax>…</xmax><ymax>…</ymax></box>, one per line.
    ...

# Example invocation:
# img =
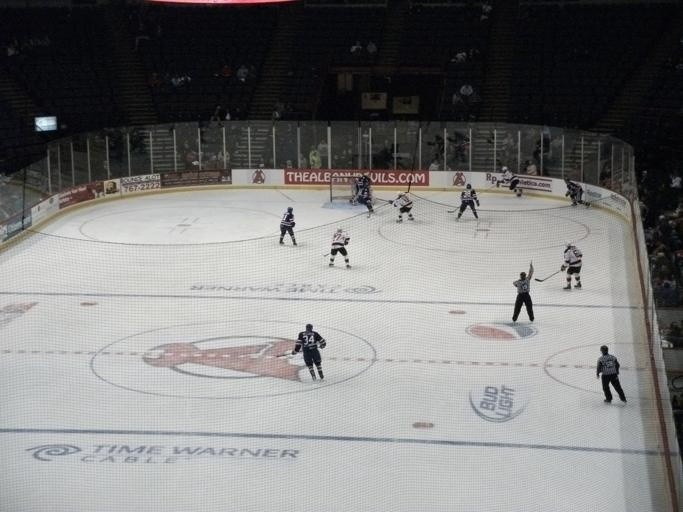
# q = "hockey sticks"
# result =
<box><xmin>448</xmin><ymin>206</ymin><xmax>461</xmax><ymax>214</ymax></box>
<box><xmin>321</xmin><ymin>252</ymin><xmax>332</xmax><ymax>258</ymax></box>
<box><xmin>276</xmin><ymin>345</ymin><xmax>321</xmax><ymax>357</ymax></box>
<box><xmin>535</xmin><ymin>269</ymin><xmax>563</xmax><ymax>282</ymax></box>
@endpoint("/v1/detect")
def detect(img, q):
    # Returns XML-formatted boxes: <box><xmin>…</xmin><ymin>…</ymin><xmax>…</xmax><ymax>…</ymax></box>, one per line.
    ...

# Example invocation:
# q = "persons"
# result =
<box><xmin>0</xmin><ymin>1</ymin><xmax>554</xmax><ymax>176</ymax></box>
<box><xmin>455</xmin><ymin>183</ymin><xmax>479</xmax><ymax>221</ymax></box>
<box><xmin>559</xmin><ymin>240</ymin><xmax>582</xmax><ymax>290</ymax></box>
<box><xmin>351</xmin><ymin>170</ymin><xmax>373</xmax><ymax>216</ymax></box>
<box><xmin>595</xmin><ymin>346</ymin><xmax>627</xmax><ymax>403</ymax></box>
<box><xmin>498</xmin><ymin>166</ymin><xmax>522</xmax><ymax>199</ymax></box>
<box><xmin>597</xmin><ymin>115</ymin><xmax>683</xmax><ymax>354</ymax></box>
<box><xmin>509</xmin><ymin>259</ymin><xmax>535</xmax><ymax>322</ymax></box>
<box><xmin>277</xmin><ymin>206</ymin><xmax>297</xmax><ymax>246</ymax></box>
<box><xmin>386</xmin><ymin>192</ymin><xmax>414</xmax><ymax>224</ymax></box>
<box><xmin>290</xmin><ymin>323</ymin><xmax>327</xmax><ymax>382</ymax></box>
<box><xmin>326</xmin><ymin>226</ymin><xmax>351</xmax><ymax>269</ymax></box>
<box><xmin>563</xmin><ymin>177</ymin><xmax>591</xmax><ymax>208</ymax></box>
<box><xmin>671</xmin><ymin>392</ymin><xmax>683</xmax><ymax>411</ymax></box>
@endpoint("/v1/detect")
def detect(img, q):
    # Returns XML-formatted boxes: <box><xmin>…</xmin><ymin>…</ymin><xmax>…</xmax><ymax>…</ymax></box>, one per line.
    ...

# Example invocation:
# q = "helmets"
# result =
<box><xmin>502</xmin><ymin>166</ymin><xmax>508</xmax><ymax>172</ymax></box>
<box><xmin>287</xmin><ymin>207</ymin><xmax>293</xmax><ymax>212</ymax></box>
<box><xmin>466</xmin><ymin>183</ymin><xmax>472</xmax><ymax>189</ymax></box>
<box><xmin>337</xmin><ymin>225</ymin><xmax>344</xmax><ymax>232</ymax></box>
<box><xmin>306</xmin><ymin>322</ymin><xmax>314</xmax><ymax>330</ymax></box>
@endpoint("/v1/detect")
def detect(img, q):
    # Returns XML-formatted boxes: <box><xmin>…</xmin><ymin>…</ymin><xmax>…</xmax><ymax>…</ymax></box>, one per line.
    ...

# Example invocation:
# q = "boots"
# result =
<box><xmin>292</xmin><ymin>239</ymin><xmax>298</xmax><ymax>245</ymax></box>
<box><xmin>407</xmin><ymin>214</ymin><xmax>413</xmax><ymax>220</ymax></box>
<box><xmin>397</xmin><ymin>215</ymin><xmax>403</xmax><ymax>222</ymax></box>
<box><xmin>328</xmin><ymin>260</ymin><xmax>335</xmax><ymax>265</ymax></box>
<box><xmin>574</xmin><ymin>281</ymin><xmax>581</xmax><ymax>288</ymax></box>
<box><xmin>343</xmin><ymin>259</ymin><xmax>351</xmax><ymax>266</ymax></box>
<box><xmin>563</xmin><ymin>283</ymin><xmax>571</xmax><ymax>289</ymax></box>
<box><xmin>316</xmin><ymin>365</ymin><xmax>324</xmax><ymax>378</ymax></box>
<box><xmin>278</xmin><ymin>238</ymin><xmax>284</xmax><ymax>244</ymax></box>
<box><xmin>308</xmin><ymin>366</ymin><xmax>317</xmax><ymax>379</ymax></box>
<box><xmin>458</xmin><ymin>213</ymin><xmax>478</xmax><ymax>218</ymax></box>
<box><xmin>571</xmin><ymin>202</ymin><xmax>590</xmax><ymax>207</ymax></box>
